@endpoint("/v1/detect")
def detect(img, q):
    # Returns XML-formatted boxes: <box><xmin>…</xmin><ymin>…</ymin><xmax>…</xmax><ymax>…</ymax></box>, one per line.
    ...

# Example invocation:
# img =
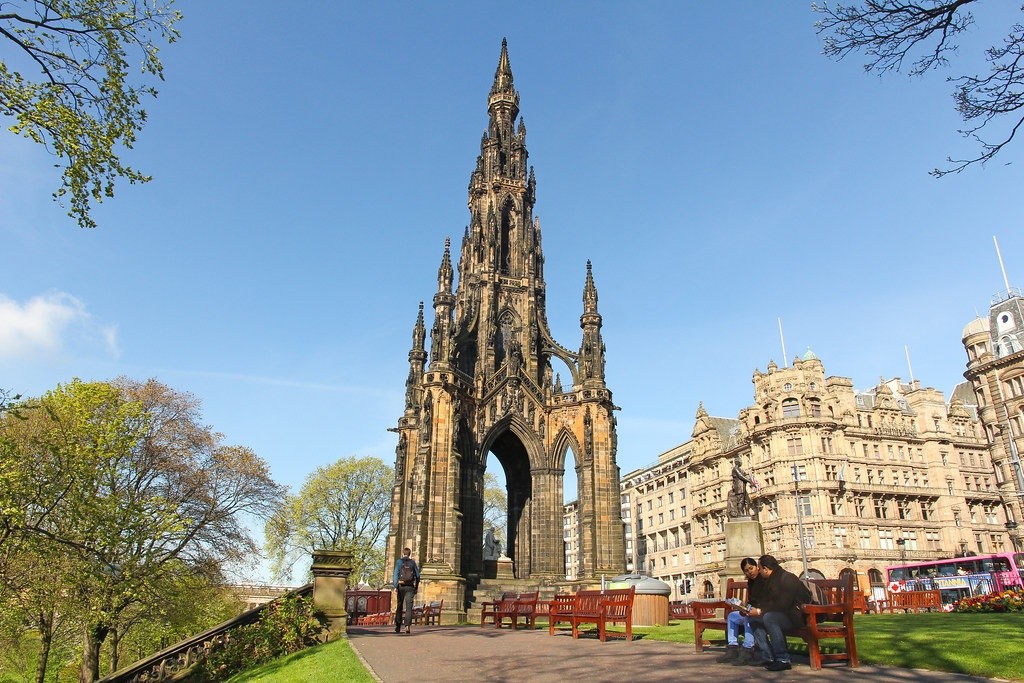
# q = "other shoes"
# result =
<box><xmin>405</xmin><ymin>626</ymin><xmax>410</xmax><ymax>633</ymax></box>
<box><xmin>748</xmin><ymin>657</ymin><xmax>773</xmax><ymax>666</ymax></box>
<box><xmin>765</xmin><ymin>661</ymin><xmax>791</xmax><ymax>672</ymax></box>
<box><xmin>395</xmin><ymin>627</ymin><xmax>401</xmax><ymax>632</ymax></box>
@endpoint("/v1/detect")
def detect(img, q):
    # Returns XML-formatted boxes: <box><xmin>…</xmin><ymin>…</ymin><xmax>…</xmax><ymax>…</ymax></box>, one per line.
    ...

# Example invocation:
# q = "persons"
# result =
<box><xmin>998</xmin><ymin>562</ymin><xmax>1011</xmax><ymax>572</ymax></box>
<box><xmin>716</xmin><ymin>554</ymin><xmax>827</xmax><ymax>672</ymax></box>
<box><xmin>392</xmin><ymin>545</ymin><xmax>421</xmax><ymax>637</ymax></box>
<box><xmin>485</xmin><ymin>527</ymin><xmax>500</xmax><ymax>556</ymax></box>
<box><xmin>728</xmin><ymin>457</ymin><xmax>758</xmax><ymax>517</ymax></box>
<box><xmin>957</xmin><ymin>566</ymin><xmax>971</xmax><ymax>576</ymax></box>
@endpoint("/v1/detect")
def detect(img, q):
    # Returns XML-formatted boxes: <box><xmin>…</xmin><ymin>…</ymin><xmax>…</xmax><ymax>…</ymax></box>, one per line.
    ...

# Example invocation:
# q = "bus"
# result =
<box><xmin>886</xmin><ymin>551</ymin><xmax>1024</xmax><ymax>613</ymax></box>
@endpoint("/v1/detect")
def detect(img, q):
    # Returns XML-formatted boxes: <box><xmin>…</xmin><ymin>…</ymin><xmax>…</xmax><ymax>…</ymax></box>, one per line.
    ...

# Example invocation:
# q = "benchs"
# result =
<box><xmin>667</xmin><ymin>589</ymin><xmax>942</xmax><ymax>621</ymax></box>
<box><xmin>358</xmin><ymin>599</ymin><xmax>444</xmax><ymax>626</ymax></box>
<box><xmin>481</xmin><ymin>584</ymin><xmax>635</xmax><ymax>642</ymax></box>
<box><xmin>689</xmin><ymin>574</ymin><xmax>858</xmax><ymax>670</ymax></box>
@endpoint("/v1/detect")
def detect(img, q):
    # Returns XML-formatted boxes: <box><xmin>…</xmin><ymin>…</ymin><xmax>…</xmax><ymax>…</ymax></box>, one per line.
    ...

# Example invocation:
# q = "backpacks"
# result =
<box><xmin>400</xmin><ymin>558</ymin><xmax>415</xmax><ymax>585</ymax></box>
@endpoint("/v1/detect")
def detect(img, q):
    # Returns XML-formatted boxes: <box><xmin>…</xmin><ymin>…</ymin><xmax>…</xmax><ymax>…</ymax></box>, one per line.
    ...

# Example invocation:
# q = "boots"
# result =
<box><xmin>716</xmin><ymin>646</ymin><xmax>739</xmax><ymax>663</ymax></box>
<box><xmin>733</xmin><ymin>648</ymin><xmax>755</xmax><ymax>666</ymax></box>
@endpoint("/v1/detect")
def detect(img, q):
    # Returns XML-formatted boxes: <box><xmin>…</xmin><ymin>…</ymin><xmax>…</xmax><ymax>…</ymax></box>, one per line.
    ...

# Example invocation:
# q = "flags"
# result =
<box><xmin>839</xmin><ymin>463</ymin><xmax>845</xmax><ymax>480</ymax></box>
<box><xmin>750</xmin><ymin>475</ymin><xmax>760</xmax><ymax>492</ymax></box>
